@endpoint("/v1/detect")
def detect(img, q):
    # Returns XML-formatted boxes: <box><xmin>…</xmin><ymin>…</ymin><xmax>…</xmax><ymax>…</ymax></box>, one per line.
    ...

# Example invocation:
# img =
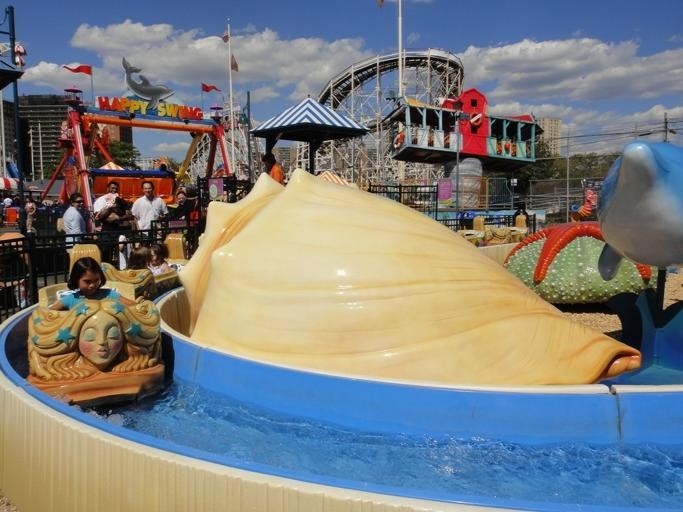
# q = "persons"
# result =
<box><xmin>128</xmin><ymin>181</ymin><xmax>170</xmax><ymax>247</ymax></box>
<box><xmin>26</xmin><ymin>299</ymin><xmax>162</xmax><ymax>368</ymax></box>
<box><xmin>49</xmin><ymin>257</ymin><xmax>139</xmax><ymax>313</ymax></box>
<box><xmin>172</xmin><ymin>192</ymin><xmax>194</xmax><ymax>221</ymax></box>
<box><xmin>261</xmin><ymin>152</ymin><xmax>283</xmax><ymax>187</ymax></box>
<box><xmin>93</xmin><ymin>182</ymin><xmax>134</xmax><ymax>265</ymax></box>
<box><xmin>64</xmin><ymin>193</ymin><xmax>87</xmax><ymax>277</ymax></box>
<box><xmin>1</xmin><ymin>194</ymin><xmax>38</xmax><ymax>235</ymax></box>
<box><xmin>125</xmin><ymin>243</ymin><xmax>170</xmax><ymax>274</ymax></box>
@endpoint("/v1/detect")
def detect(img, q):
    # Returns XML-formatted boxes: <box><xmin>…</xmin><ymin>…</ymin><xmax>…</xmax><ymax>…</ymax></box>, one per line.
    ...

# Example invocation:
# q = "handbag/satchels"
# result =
<box><xmin>56</xmin><ymin>218</ymin><xmax>64</xmax><ymax>232</ymax></box>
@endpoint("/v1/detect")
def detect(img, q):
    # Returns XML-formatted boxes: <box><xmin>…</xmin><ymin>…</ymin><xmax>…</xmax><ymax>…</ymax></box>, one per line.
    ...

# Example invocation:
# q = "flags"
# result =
<box><xmin>202</xmin><ymin>83</ymin><xmax>222</xmax><ymax>93</ymax></box>
<box><xmin>231</xmin><ymin>55</ymin><xmax>238</xmax><ymax>72</ymax></box>
<box><xmin>222</xmin><ymin>30</ymin><xmax>228</xmax><ymax>42</ymax></box>
<box><xmin>63</xmin><ymin>65</ymin><xmax>91</xmax><ymax>75</ymax></box>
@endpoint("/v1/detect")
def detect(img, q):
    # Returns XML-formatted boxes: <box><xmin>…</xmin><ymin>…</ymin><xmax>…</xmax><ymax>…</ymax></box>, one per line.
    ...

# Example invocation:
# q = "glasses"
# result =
<box><xmin>73</xmin><ymin>200</ymin><xmax>83</xmax><ymax>203</ymax></box>
